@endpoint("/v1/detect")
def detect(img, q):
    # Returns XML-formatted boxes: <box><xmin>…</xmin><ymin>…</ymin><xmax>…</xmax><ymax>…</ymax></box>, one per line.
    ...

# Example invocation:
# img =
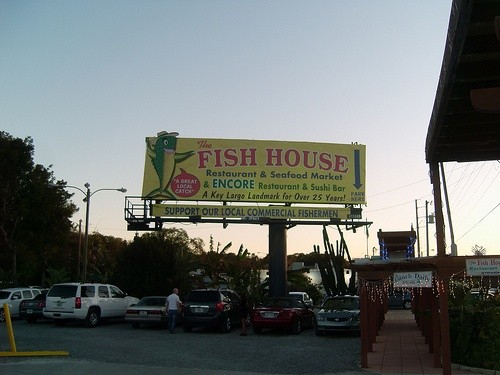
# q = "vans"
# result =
<box><xmin>0</xmin><ymin>287</ymin><xmax>41</xmax><ymax>322</ymax></box>
<box><xmin>42</xmin><ymin>281</ymin><xmax>140</xmax><ymax>328</ymax></box>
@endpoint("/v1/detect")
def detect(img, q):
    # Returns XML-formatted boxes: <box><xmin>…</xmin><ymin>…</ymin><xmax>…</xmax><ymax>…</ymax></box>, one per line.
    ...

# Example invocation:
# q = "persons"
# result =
<box><xmin>164</xmin><ymin>289</ymin><xmax>182</xmax><ymax>332</ymax></box>
<box><xmin>236</xmin><ymin>288</ymin><xmax>249</xmax><ymax>336</ymax></box>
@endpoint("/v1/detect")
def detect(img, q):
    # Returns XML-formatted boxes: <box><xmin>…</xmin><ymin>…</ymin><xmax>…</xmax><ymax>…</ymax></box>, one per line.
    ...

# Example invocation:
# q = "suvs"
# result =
<box><xmin>180</xmin><ymin>287</ymin><xmax>245</xmax><ymax>333</ymax></box>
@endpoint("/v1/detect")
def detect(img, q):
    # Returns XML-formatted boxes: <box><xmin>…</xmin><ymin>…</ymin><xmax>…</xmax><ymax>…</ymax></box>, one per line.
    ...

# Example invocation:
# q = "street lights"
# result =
<box><xmin>46</xmin><ymin>182</ymin><xmax>127</xmax><ymax>284</ymax></box>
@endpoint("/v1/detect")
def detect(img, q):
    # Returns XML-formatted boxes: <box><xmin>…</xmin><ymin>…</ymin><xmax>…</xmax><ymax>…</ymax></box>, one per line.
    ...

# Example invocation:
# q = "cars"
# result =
<box><xmin>315</xmin><ymin>293</ymin><xmax>361</xmax><ymax>335</ymax></box>
<box><xmin>250</xmin><ymin>296</ymin><xmax>316</xmax><ymax>336</ymax></box>
<box><xmin>123</xmin><ymin>295</ymin><xmax>171</xmax><ymax>330</ymax></box>
<box><xmin>288</xmin><ymin>291</ymin><xmax>314</xmax><ymax>306</ymax></box>
<box><xmin>18</xmin><ymin>290</ymin><xmax>49</xmax><ymax>324</ymax></box>
<box><xmin>388</xmin><ymin>287</ymin><xmax>500</xmax><ymax>309</ymax></box>
